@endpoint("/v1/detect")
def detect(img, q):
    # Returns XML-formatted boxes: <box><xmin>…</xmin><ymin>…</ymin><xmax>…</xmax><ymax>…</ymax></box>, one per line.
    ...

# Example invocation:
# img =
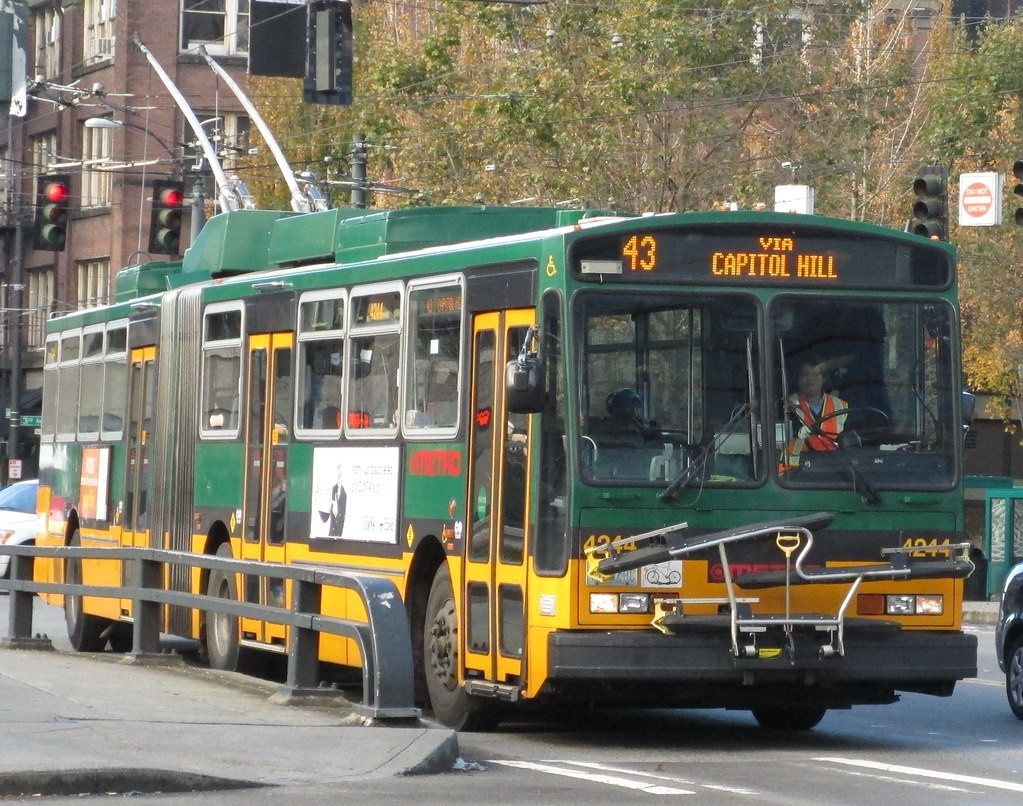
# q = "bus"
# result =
<box><xmin>34</xmin><ymin>29</ymin><xmax>977</xmax><ymax>731</ymax></box>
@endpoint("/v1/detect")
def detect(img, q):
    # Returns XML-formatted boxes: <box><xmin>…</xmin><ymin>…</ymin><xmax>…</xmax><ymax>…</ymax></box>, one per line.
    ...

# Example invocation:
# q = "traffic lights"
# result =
<box><xmin>1012</xmin><ymin>160</ymin><xmax>1023</xmax><ymax>229</ymax></box>
<box><xmin>149</xmin><ymin>180</ymin><xmax>186</xmax><ymax>255</ymax></box>
<box><xmin>32</xmin><ymin>173</ymin><xmax>71</xmax><ymax>251</ymax></box>
<box><xmin>912</xmin><ymin>163</ymin><xmax>951</xmax><ymax>246</ymax></box>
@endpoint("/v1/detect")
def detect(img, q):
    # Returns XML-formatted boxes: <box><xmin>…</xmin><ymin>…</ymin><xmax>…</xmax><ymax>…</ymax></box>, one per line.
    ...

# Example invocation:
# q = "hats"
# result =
<box><xmin>605</xmin><ymin>388</ymin><xmax>641</xmax><ymax>414</ymax></box>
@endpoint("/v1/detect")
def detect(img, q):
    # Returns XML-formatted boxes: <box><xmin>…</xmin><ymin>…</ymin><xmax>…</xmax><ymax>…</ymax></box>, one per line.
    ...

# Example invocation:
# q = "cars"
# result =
<box><xmin>993</xmin><ymin>561</ymin><xmax>1022</xmax><ymax>723</ymax></box>
<box><xmin>0</xmin><ymin>478</ymin><xmax>41</xmax><ymax>596</ymax></box>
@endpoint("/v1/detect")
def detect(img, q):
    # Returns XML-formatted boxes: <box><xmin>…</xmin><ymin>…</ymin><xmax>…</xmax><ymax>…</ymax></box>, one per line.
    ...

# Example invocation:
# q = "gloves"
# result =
<box><xmin>798</xmin><ymin>425</ymin><xmax>811</xmax><ymax>440</ymax></box>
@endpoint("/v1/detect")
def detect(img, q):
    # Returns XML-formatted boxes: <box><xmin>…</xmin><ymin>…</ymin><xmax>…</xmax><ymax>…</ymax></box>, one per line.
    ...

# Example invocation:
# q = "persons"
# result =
<box><xmin>316</xmin><ymin>459</ymin><xmax>346</xmax><ymax>538</ymax></box>
<box><xmin>599</xmin><ymin>388</ymin><xmax>665</xmax><ymax>450</ymax></box>
<box><xmin>775</xmin><ymin>354</ymin><xmax>858</xmax><ymax>479</ymax></box>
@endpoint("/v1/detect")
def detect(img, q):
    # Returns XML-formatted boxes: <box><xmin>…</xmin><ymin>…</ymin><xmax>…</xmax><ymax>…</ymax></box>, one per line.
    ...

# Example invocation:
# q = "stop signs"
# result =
<box><xmin>959</xmin><ymin>170</ymin><xmax>1000</xmax><ymax>227</ymax></box>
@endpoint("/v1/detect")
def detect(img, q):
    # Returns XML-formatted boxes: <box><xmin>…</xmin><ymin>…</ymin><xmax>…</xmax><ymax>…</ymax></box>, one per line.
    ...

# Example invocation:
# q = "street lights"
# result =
<box><xmin>83</xmin><ymin>117</ymin><xmax>204</xmax><ymax>247</ymax></box>
